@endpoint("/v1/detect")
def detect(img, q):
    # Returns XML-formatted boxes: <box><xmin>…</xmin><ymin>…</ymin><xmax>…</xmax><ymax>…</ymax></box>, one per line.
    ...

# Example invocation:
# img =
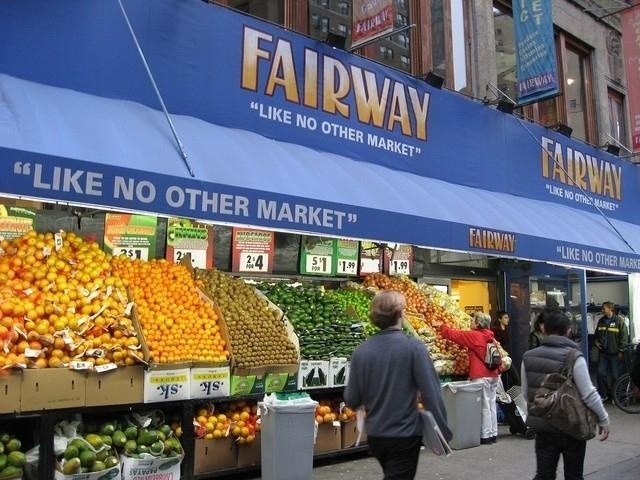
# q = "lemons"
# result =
<box><xmin>195</xmin><ymin>401</ymin><xmax>356</xmax><ymax>444</ymax></box>
<box><xmin>0</xmin><ymin>226</ymin><xmax>144</xmax><ymax>371</ymax></box>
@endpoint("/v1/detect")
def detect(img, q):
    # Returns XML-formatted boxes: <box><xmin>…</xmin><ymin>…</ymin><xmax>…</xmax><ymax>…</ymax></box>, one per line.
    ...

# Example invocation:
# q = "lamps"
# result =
<box><xmin>496</xmin><ymin>99</ymin><xmax>514</xmax><ymax>114</ymax></box>
<box><xmin>326</xmin><ymin>28</ymin><xmax>345</xmax><ymax>48</ymax></box>
<box><xmin>607</xmin><ymin>143</ymin><xmax>620</xmax><ymax>156</ymax></box>
<box><xmin>557</xmin><ymin>123</ymin><xmax>573</xmax><ymax>138</ymax></box>
<box><xmin>427</xmin><ymin>71</ymin><xmax>445</xmax><ymax>89</ymax></box>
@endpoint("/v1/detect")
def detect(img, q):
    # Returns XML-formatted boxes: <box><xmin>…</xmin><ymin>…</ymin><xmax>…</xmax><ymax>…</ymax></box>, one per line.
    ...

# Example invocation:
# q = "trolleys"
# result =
<box><xmin>497</xmin><ymin>363</ymin><xmax>537</xmax><ymax>440</ymax></box>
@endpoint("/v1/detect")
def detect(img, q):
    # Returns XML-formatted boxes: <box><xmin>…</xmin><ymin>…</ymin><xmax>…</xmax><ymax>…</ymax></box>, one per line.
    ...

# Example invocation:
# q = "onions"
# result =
<box><xmin>367</xmin><ymin>270</ymin><xmax>471</xmax><ymax>375</ymax></box>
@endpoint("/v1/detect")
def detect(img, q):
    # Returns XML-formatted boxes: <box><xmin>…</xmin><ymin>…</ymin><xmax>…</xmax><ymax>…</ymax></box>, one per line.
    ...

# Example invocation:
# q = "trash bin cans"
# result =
<box><xmin>257</xmin><ymin>397</ymin><xmax>317</xmax><ymax>480</ymax></box>
<box><xmin>440</xmin><ymin>379</ymin><xmax>484</xmax><ymax>451</ymax></box>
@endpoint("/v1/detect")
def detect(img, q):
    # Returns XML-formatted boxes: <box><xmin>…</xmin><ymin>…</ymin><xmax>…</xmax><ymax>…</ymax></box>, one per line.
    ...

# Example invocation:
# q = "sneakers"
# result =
<box><xmin>480</xmin><ymin>436</ymin><xmax>497</xmax><ymax>445</ymax></box>
<box><xmin>600</xmin><ymin>394</ymin><xmax>609</xmax><ymax>405</ymax></box>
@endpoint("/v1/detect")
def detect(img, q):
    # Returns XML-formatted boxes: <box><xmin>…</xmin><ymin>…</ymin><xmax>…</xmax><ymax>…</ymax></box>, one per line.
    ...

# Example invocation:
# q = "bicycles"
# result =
<box><xmin>614</xmin><ymin>343</ymin><xmax>640</xmax><ymax>413</ymax></box>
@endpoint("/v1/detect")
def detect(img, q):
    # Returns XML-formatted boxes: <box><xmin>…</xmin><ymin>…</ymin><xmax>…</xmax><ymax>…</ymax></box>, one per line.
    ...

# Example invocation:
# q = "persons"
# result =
<box><xmin>436</xmin><ymin>311</ymin><xmax>501</xmax><ymax>446</ymax></box>
<box><xmin>493</xmin><ymin>311</ymin><xmax>519</xmax><ymax>391</ymax></box>
<box><xmin>528</xmin><ymin>312</ymin><xmax>548</xmax><ymax>350</ymax></box>
<box><xmin>593</xmin><ymin>300</ymin><xmax>628</xmax><ymax>404</ymax></box>
<box><xmin>517</xmin><ymin>309</ymin><xmax>610</xmax><ymax>480</ymax></box>
<box><xmin>342</xmin><ymin>290</ymin><xmax>452</xmax><ymax>479</ymax></box>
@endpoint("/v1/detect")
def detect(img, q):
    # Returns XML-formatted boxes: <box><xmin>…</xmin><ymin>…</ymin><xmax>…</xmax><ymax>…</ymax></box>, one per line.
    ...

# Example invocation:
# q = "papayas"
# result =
<box><xmin>0</xmin><ymin>417</ymin><xmax>180</xmax><ymax>480</ymax></box>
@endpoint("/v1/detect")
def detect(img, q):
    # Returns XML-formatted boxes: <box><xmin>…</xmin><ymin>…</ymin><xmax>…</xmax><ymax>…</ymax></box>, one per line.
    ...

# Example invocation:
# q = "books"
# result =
<box><xmin>420</xmin><ymin>411</ymin><xmax>452</xmax><ymax>457</ymax></box>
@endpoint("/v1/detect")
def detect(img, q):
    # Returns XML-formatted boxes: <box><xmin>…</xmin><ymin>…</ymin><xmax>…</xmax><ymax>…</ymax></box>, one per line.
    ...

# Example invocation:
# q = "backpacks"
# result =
<box><xmin>474</xmin><ymin>330</ymin><xmax>501</xmax><ymax>371</ymax></box>
<box><xmin>495</xmin><ymin>339</ymin><xmax>513</xmax><ymax>373</ymax></box>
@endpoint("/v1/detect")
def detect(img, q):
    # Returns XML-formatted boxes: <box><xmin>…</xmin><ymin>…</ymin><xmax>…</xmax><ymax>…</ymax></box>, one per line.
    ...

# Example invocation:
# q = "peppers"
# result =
<box><xmin>329</xmin><ymin>286</ymin><xmax>413</xmax><ymax>337</ymax></box>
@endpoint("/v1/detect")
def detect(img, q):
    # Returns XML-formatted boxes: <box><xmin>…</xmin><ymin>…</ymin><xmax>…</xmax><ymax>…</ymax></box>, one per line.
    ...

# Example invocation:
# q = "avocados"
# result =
<box><xmin>261</xmin><ymin>278</ymin><xmax>367</xmax><ymax>360</ymax></box>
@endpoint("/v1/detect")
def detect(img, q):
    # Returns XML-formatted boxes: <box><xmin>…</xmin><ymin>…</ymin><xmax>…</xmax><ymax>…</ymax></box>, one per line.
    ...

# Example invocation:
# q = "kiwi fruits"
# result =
<box><xmin>195</xmin><ymin>266</ymin><xmax>298</xmax><ymax>366</ymax></box>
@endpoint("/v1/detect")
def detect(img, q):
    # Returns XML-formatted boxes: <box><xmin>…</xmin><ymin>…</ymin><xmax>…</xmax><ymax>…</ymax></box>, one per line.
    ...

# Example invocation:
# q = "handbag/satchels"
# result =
<box><xmin>523</xmin><ymin>349</ymin><xmax>597</xmax><ymax>442</ymax></box>
